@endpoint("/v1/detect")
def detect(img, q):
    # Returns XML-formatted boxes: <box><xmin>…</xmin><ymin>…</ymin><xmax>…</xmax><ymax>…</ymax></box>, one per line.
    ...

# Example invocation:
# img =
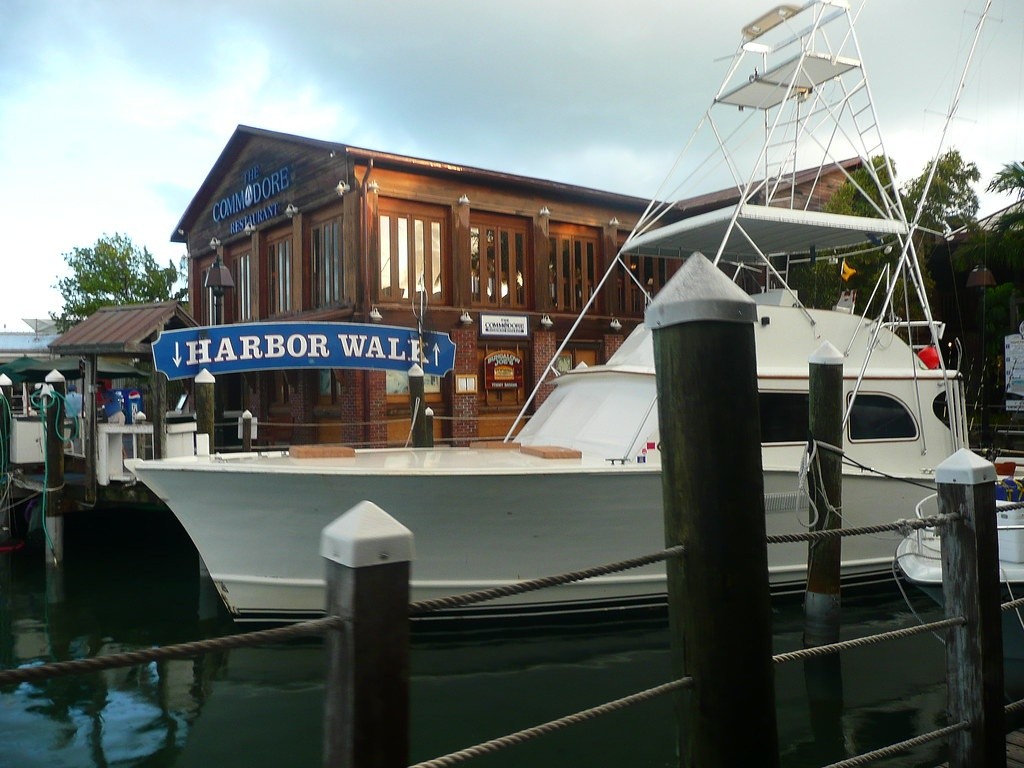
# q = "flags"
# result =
<box><xmin>842</xmin><ymin>262</ymin><xmax>856</xmax><ymax>282</ymax></box>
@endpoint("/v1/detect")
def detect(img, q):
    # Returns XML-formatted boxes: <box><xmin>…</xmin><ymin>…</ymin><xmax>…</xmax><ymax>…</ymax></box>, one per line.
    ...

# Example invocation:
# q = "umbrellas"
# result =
<box><xmin>18</xmin><ymin>356</ymin><xmax>150</xmax><ymax>419</ymax></box>
<box><xmin>0</xmin><ymin>357</ymin><xmax>43</xmax><ymax>417</ymax></box>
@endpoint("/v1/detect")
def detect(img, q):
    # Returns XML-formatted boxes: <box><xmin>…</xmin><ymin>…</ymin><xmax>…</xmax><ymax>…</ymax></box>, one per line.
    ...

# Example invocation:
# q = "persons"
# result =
<box><xmin>97</xmin><ymin>382</ymin><xmax>128</xmax><ymax>459</ymax></box>
<box><xmin>64</xmin><ymin>385</ymin><xmax>85</xmax><ymax>418</ymax></box>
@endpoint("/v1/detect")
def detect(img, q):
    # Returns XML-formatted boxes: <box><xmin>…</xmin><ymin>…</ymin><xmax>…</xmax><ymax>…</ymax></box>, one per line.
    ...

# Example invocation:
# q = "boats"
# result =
<box><xmin>124</xmin><ymin>0</ymin><xmax>969</xmax><ymax>639</ymax></box>
<box><xmin>894</xmin><ymin>489</ymin><xmax>1024</xmax><ymax>658</ymax></box>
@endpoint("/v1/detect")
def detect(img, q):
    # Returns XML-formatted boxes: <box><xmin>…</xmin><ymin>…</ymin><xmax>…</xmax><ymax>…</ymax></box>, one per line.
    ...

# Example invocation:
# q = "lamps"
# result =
<box><xmin>284</xmin><ymin>204</ymin><xmax>298</xmax><ymax>217</ymax></box>
<box><xmin>371</xmin><ymin>309</ymin><xmax>383</xmax><ymax>323</ymax></box>
<box><xmin>610</xmin><ymin>319</ymin><xmax>622</xmax><ymax>329</ymax></box>
<box><xmin>243</xmin><ymin>222</ymin><xmax>256</xmax><ymax>234</ymax></box>
<box><xmin>208</xmin><ymin>236</ymin><xmax>221</xmax><ymax>250</ymax></box>
<box><xmin>608</xmin><ymin>217</ymin><xmax>620</xmax><ymax>226</ymax></box>
<box><xmin>541</xmin><ymin>314</ymin><xmax>553</xmax><ymax>328</ymax></box>
<box><xmin>367</xmin><ymin>180</ymin><xmax>380</xmax><ymax>194</ymax></box>
<box><xmin>539</xmin><ymin>206</ymin><xmax>550</xmax><ymax>216</ymax></box>
<box><xmin>334</xmin><ymin>181</ymin><xmax>350</xmax><ymax>196</ymax></box>
<box><xmin>460</xmin><ymin>312</ymin><xmax>473</xmax><ymax>324</ymax></box>
<box><xmin>459</xmin><ymin>193</ymin><xmax>470</xmax><ymax>204</ymax></box>
